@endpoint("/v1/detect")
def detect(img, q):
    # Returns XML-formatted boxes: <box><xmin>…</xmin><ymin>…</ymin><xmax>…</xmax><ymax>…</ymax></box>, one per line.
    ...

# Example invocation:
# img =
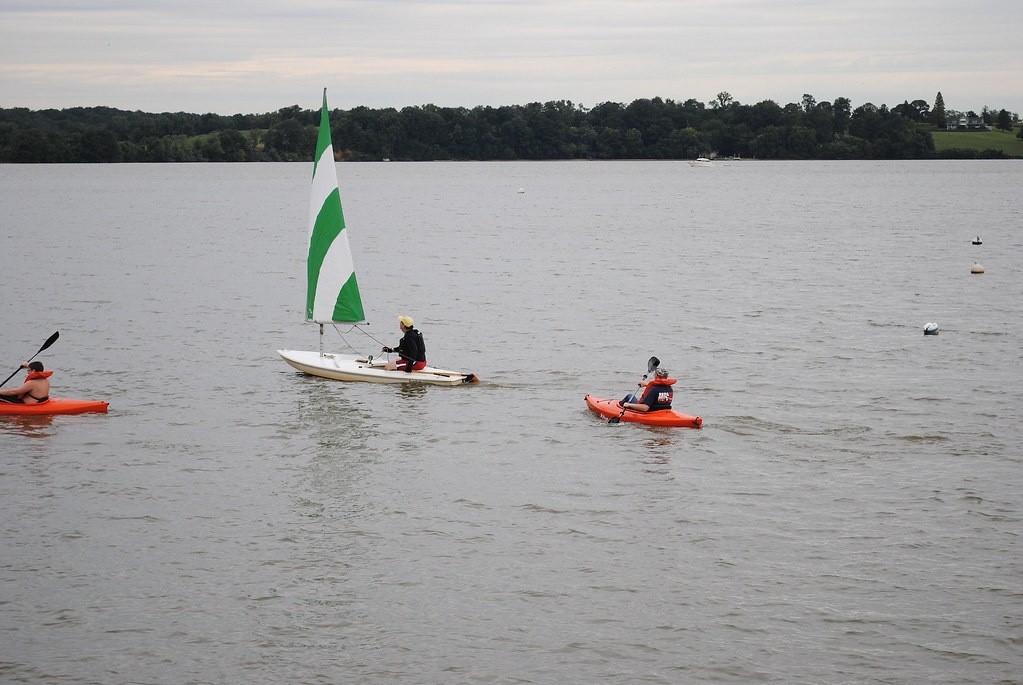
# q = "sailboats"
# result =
<box><xmin>275</xmin><ymin>85</ymin><xmax>476</xmax><ymax>385</ymax></box>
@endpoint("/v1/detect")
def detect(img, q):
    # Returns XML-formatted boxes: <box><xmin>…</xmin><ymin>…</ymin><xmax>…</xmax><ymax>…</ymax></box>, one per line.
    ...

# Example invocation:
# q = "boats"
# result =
<box><xmin>585</xmin><ymin>394</ymin><xmax>702</xmax><ymax>428</ymax></box>
<box><xmin>1</xmin><ymin>393</ymin><xmax>110</xmax><ymax>418</ymax></box>
<box><xmin>689</xmin><ymin>157</ymin><xmax>712</xmax><ymax>167</ymax></box>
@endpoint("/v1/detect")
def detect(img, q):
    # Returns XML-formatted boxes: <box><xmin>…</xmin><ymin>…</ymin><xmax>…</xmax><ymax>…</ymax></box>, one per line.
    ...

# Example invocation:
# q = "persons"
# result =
<box><xmin>382</xmin><ymin>315</ymin><xmax>426</xmax><ymax>372</ymax></box>
<box><xmin>619</xmin><ymin>368</ymin><xmax>674</xmax><ymax>411</ymax></box>
<box><xmin>0</xmin><ymin>361</ymin><xmax>50</xmax><ymax>404</ymax></box>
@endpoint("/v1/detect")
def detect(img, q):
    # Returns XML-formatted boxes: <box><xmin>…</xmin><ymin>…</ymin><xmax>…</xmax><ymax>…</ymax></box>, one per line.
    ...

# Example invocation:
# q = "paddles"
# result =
<box><xmin>607</xmin><ymin>356</ymin><xmax>660</xmax><ymax>424</ymax></box>
<box><xmin>0</xmin><ymin>331</ymin><xmax>60</xmax><ymax>389</ymax></box>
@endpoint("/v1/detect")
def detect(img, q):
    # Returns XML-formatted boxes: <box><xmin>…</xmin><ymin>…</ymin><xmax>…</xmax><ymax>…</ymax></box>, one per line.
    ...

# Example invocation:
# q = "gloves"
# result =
<box><xmin>405</xmin><ymin>364</ymin><xmax>412</xmax><ymax>372</ymax></box>
<box><xmin>383</xmin><ymin>347</ymin><xmax>392</xmax><ymax>353</ymax></box>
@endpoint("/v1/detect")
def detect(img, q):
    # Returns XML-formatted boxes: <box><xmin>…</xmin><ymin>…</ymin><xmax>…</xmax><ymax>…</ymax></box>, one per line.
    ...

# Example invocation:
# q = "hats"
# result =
<box><xmin>655</xmin><ymin>368</ymin><xmax>669</xmax><ymax>377</ymax></box>
<box><xmin>398</xmin><ymin>315</ymin><xmax>414</xmax><ymax>328</ymax></box>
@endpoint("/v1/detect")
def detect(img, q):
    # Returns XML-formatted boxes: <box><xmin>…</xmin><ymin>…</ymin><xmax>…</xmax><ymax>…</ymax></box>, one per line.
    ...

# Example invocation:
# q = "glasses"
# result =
<box><xmin>27</xmin><ymin>369</ymin><xmax>33</xmax><ymax>372</ymax></box>
